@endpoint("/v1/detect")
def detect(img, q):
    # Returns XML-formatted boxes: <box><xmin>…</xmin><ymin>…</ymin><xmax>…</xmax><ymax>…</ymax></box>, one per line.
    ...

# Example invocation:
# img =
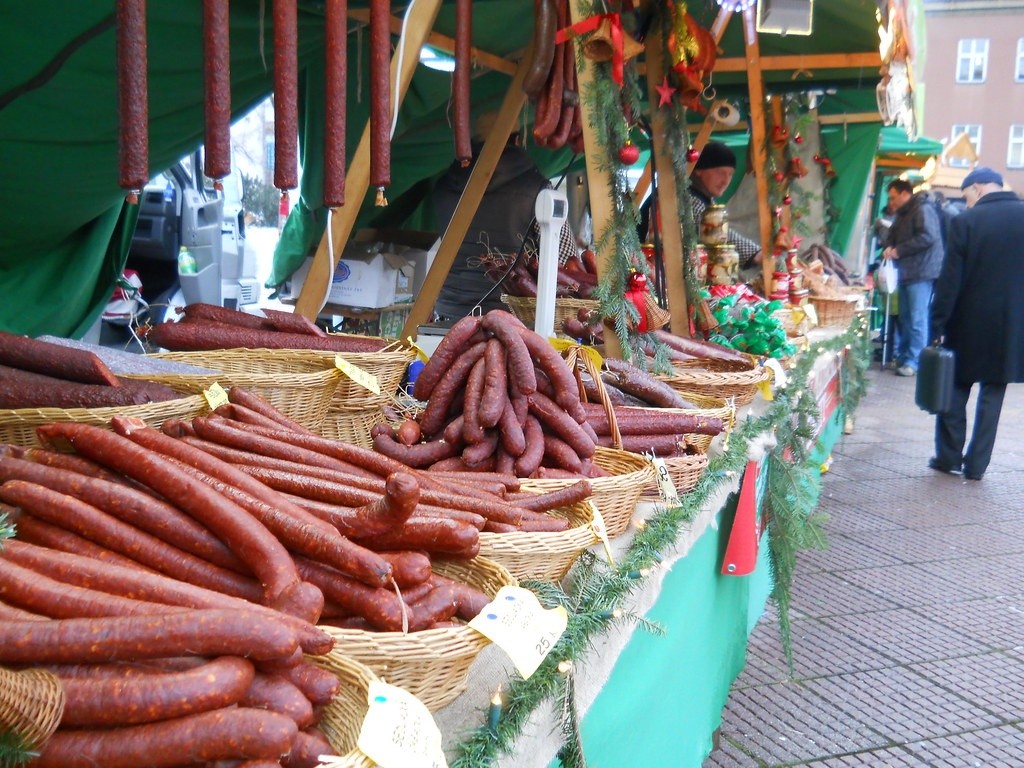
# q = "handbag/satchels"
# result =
<box><xmin>878</xmin><ymin>253</ymin><xmax>899</xmax><ymax>294</ymax></box>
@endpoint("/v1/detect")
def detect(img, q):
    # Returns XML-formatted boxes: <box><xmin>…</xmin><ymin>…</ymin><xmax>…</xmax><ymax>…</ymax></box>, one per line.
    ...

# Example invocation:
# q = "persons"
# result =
<box><xmin>640</xmin><ymin>141</ymin><xmax>765</xmax><ymax>270</ymax></box>
<box><xmin>925</xmin><ymin>167</ymin><xmax>1024</xmax><ymax>479</ymax></box>
<box><xmin>370</xmin><ymin>109</ymin><xmax>578</xmax><ymax>318</ymax></box>
<box><xmin>916</xmin><ymin>188</ymin><xmax>959</xmax><ymax>232</ymax></box>
<box><xmin>872</xmin><ymin>180</ymin><xmax>942</xmax><ymax>376</ymax></box>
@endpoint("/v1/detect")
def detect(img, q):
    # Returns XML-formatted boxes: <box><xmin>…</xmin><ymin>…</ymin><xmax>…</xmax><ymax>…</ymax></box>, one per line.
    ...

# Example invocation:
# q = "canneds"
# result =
<box><xmin>769</xmin><ymin>248</ymin><xmax>810</xmax><ymax>308</ymax></box>
<box><xmin>637</xmin><ymin>203</ymin><xmax>740</xmax><ymax>286</ymax></box>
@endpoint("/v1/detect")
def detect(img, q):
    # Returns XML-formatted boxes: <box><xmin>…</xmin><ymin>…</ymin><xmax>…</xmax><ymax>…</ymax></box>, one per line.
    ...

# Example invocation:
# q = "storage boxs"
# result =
<box><xmin>286</xmin><ymin>248</ymin><xmax>416</xmax><ymax>308</ymax></box>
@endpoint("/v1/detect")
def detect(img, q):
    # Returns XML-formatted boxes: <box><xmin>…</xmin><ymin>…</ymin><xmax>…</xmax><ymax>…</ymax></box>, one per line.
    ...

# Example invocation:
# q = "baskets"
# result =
<box><xmin>772</xmin><ymin>307</ymin><xmax>809</xmax><ymax>337</ymax></box>
<box><xmin>811</xmin><ymin>294</ymin><xmax>856</xmax><ymax>330</ymax></box>
<box><xmin>0</xmin><ymin>292</ymin><xmax>773</xmax><ymax>767</ymax></box>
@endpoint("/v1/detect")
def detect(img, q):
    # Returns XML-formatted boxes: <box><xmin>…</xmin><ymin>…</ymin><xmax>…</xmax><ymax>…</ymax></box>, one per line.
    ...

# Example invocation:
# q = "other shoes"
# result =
<box><xmin>897</xmin><ymin>361</ymin><xmax>916</xmax><ymax>379</ymax></box>
<box><xmin>929</xmin><ymin>453</ymin><xmax>963</xmax><ymax>471</ymax></box>
<box><xmin>968</xmin><ymin>471</ymin><xmax>983</xmax><ymax>481</ymax></box>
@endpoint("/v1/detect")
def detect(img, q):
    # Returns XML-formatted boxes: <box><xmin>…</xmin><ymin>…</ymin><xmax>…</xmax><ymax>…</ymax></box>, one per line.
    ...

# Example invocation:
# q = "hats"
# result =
<box><xmin>961</xmin><ymin>166</ymin><xmax>1004</xmax><ymax>188</ymax></box>
<box><xmin>697</xmin><ymin>142</ymin><xmax>737</xmax><ymax>171</ymax></box>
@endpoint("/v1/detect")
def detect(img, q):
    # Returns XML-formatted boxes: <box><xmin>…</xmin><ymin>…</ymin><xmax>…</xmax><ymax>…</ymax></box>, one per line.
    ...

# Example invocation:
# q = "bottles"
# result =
<box><xmin>640</xmin><ymin>204</ymin><xmax>740</xmax><ymax>288</ymax></box>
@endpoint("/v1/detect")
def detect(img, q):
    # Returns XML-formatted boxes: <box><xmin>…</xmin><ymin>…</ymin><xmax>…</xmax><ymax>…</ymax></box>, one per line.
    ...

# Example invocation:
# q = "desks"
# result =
<box><xmin>426</xmin><ymin>316</ymin><xmax>873</xmax><ymax>767</ymax></box>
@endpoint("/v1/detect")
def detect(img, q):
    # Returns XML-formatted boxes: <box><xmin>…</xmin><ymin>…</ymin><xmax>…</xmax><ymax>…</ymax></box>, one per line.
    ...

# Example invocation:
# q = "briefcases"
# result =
<box><xmin>915</xmin><ymin>335</ymin><xmax>955</xmax><ymax>416</ymax></box>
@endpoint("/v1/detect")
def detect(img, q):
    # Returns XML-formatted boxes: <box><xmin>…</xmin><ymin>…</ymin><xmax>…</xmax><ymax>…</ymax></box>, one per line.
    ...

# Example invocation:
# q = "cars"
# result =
<box><xmin>277</xmin><ymin>127</ymin><xmax>303</xmax><ymax>304</ymax></box>
<box><xmin>99</xmin><ymin>136</ymin><xmax>260</xmax><ymax>353</ymax></box>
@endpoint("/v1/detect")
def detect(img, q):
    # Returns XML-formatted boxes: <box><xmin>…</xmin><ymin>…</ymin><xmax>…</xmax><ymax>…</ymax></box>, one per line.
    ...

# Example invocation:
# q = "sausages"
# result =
<box><xmin>115</xmin><ymin>0</ymin><xmax>633</xmax><ymax>214</ymax></box>
<box><xmin>479</xmin><ymin>249</ymin><xmax>601</xmax><ymax>300</ymax></box>
<box><xmin>0</xmin><ymin>307</ymin><xmax>756</xmax><ymax>768</ymax></box>
